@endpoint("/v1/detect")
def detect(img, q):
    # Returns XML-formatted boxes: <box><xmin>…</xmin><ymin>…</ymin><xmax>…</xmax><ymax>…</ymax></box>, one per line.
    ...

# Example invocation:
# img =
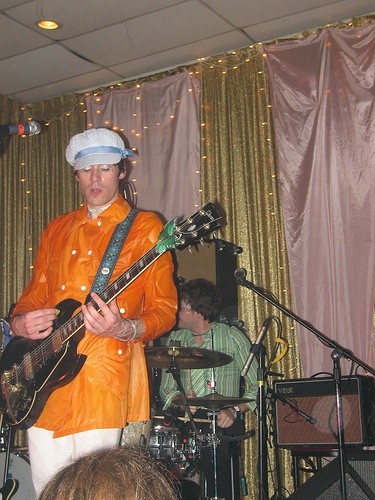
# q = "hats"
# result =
<box><xmin>66</xmin><ymin>128</ymin><xmax>134</xmax><ymax>173</ymax></box>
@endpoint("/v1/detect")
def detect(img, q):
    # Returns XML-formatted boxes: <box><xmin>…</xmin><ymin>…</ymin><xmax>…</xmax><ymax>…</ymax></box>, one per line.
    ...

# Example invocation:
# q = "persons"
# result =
<box><xmin>158</xmin><ymin>279</ymin><xmax>268</xmax><ymax>500</ymax></box>
<box><xmin>7</xmin><ymin>129</ymin><xmax>179</xmax><ymax>495</ymax></box>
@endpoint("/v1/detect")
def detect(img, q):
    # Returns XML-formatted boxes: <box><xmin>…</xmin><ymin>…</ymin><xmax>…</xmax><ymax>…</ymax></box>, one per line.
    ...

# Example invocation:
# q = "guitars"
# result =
<box><xmin>0</xmin><ymin>201</ymin><xmax>224</xmax><ymax>431</ymax></box>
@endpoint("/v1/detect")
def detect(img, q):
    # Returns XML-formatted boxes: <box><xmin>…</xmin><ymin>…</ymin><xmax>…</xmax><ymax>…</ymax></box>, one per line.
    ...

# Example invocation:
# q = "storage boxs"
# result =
<box><xmin>272</xmin><ymin>374</ymin><xmax>375</xmax><ymax>450</ymax></box>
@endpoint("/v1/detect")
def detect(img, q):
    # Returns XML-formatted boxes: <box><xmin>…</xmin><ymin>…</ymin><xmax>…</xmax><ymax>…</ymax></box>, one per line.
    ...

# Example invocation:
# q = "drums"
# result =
<box><xmin>141</xmin><ymin>415</ymin><xmax>217</xmax><ymax>478</ymax></box>
<box><xmin>0</xmin><ymin>450</ymin><xmax>37</xmax><ymax>500</ymax></box>
<box><xmin>0</xmin><ymin>428</ymin><xmax>29</xmax><ymax>451</ymax></box>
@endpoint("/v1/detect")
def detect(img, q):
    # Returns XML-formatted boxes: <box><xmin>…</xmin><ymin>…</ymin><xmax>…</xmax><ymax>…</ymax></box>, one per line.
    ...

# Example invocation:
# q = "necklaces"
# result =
<box><xmin>191</xmin><ymin>330</ymin><xmax>210</xmax><ymax>336</ymax></box>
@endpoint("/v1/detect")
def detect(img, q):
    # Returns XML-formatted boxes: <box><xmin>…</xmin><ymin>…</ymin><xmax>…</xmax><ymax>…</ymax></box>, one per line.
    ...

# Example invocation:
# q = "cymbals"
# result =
<box><xmin>144</xmin><ymin>346</ymin><xmax>233</xmax><ymax>369</ymax></box>
<box><xmin>171</xmin><ymin>393</ymin><xmax>256</xmax><ymax>408</ymax></box>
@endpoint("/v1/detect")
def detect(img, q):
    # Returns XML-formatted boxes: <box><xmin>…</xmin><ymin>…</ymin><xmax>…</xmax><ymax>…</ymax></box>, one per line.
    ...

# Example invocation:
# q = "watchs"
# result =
<box><xmin>228</xmin><ymin>405</ymin><xmax>241</xmax><ymax>419</ymax></box>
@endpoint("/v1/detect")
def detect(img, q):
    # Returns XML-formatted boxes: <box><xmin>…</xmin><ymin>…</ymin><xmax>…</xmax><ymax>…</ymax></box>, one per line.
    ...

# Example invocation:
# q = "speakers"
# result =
<box><xmin>272</xmin><ymin>375</ymin><xmax>374</xmax><ymax>447</ymax></box>
<box><xmin>168</xmin><ymin>238</ymin><xmax>239</xmax><ymax>328</ymax></box>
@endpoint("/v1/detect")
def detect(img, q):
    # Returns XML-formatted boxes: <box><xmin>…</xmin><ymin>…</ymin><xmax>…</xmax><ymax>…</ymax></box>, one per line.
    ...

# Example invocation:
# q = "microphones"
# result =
<box><xmin>291</xmin><ymin>406</ymin><xmax>315</xmax><ymax>424</ymax></box>
<box><xmin>241</xmin><ymin>317</ymin><xmax>272</xmax><ymax>376</ymax></box>
<box><xmin>162</xmin><ymin>407</ymin><xmax>173</xmax><ymax>426</ymax></box>
<box><xmin>234</xmin><ymin>268</ymin><xmax>246</xmax><ymax>285</ymax></box>
<box><xmin>0</xmin><ymin>120</ymin><xmax>41</xmax><ymax>137</ymax></box>
